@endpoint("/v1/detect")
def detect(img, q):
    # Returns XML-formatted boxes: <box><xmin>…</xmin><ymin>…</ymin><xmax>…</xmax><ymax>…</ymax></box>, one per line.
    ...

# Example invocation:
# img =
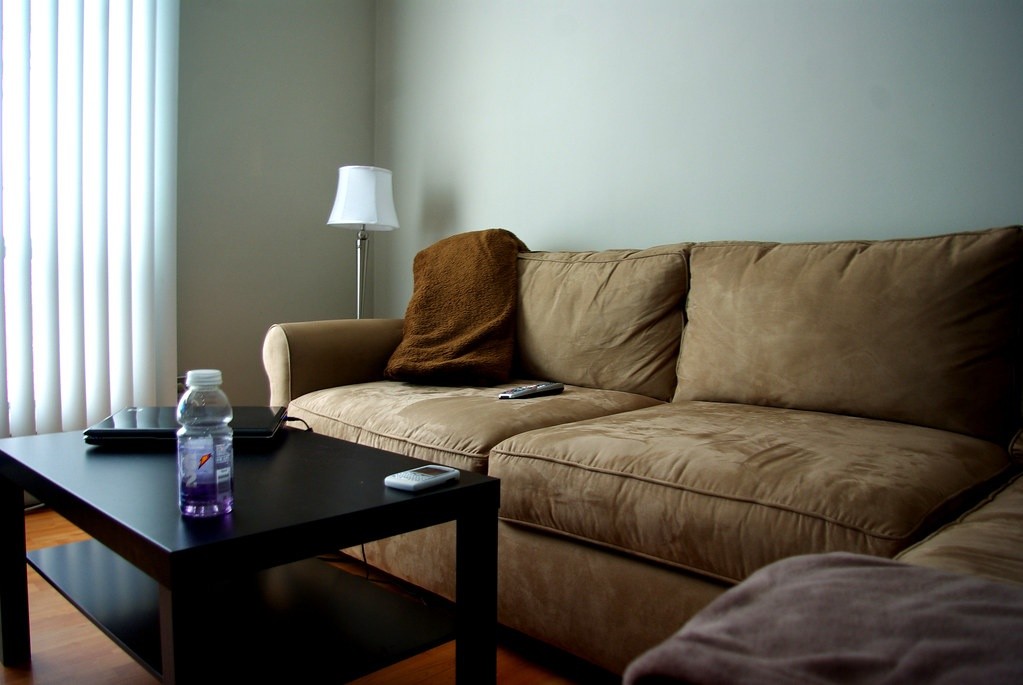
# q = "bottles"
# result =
<box><xmin>176</xmin><ymin>369</ymin><xmax>234</xmax><ymax>518</ymax></box>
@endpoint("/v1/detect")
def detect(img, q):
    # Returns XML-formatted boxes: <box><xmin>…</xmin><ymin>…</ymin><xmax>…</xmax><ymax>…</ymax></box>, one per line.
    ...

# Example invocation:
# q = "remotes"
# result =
<box><xmin>499</xmin><ymin>382</ymin><xmax>565</xmax><ymax>399</ymax></box>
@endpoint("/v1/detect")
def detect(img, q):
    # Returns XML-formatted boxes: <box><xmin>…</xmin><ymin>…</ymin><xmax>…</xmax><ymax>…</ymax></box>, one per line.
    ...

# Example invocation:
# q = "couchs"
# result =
<box><xmin>263</xmin><ymin>226</ymin><xmax>1022</xmax><ymax>684</ymax></box>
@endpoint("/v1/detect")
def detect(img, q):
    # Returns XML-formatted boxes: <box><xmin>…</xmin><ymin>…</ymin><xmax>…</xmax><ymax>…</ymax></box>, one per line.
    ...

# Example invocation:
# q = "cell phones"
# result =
<box><xmin>384</xmin><ymin>464</ymin><xmax>460</xmax><ymax>492</ymax></box>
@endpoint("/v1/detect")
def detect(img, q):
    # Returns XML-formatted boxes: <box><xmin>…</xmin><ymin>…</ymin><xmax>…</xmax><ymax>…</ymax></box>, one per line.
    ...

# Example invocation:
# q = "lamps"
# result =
<box><xmin>328</xmin><ymin>165</ymin><xmax>401</xmax><ymax>320</ymax></box>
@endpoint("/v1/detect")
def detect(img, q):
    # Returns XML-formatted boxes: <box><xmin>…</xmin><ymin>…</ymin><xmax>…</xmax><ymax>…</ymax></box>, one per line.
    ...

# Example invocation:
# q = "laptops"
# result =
<box><xmin>83</xmin><ymin>405</ymin><xmax>288</xmax><ymax>446</ymax></box>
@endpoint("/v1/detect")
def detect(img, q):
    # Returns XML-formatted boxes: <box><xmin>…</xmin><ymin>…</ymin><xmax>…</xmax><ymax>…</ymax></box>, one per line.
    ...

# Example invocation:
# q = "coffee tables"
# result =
<box><xmin>0</xmin><ymin>425</ymin><xmax>502</xmax><ymax>685</ymax></box>
